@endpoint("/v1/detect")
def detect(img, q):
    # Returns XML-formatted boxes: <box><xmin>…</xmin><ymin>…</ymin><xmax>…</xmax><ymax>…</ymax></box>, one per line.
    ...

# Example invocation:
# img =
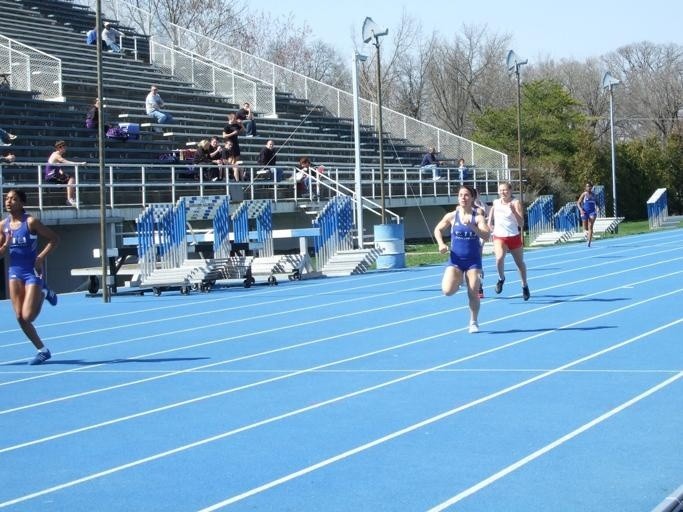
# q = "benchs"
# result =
<box><xmin>276</xmin><ymin>92</ymin><xmax>503</xmax><ymax>189</ymax></box>
<box><xmin>102</xmin><ymin>15</ymin><xmax>278</xmax><ymax>189</ymax></box>
<box><xmin>1</xmin><ymin>2</ymin><xmax>97</xmax><ymax>195</ymax></box>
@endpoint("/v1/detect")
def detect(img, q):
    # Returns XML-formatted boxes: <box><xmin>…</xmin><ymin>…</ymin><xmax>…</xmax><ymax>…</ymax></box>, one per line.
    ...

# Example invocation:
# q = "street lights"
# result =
<box><xmin>506</xmin><ymin>49</ymin><xmax>527</xmax><ymax>247</ymax></box>
<box><xmin>360</xmin><ymin>13</ymin><xmax>389</xmax><ymax>224</ymax></box>
<box><xmin>601</xmin><ymin>71</ymin><xmax>623</xmax><ymax>234</ymax></box>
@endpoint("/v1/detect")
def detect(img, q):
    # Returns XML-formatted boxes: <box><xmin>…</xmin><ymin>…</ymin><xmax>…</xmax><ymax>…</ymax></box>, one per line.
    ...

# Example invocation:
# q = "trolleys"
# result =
<box><xmin>68</xmin><ymin>193</ymin><xmax>317</xmax><ymax>302</ymax></box>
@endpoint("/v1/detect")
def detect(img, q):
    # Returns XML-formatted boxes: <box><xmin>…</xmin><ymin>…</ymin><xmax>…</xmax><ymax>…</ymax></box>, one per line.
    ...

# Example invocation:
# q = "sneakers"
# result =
<box><xmin>478</xmin><ymin>282</ymin><xmax>485</xmax><ymax>299</ymax></box>
<box><xmin>42</xmin><ymin>281</ymin><xmax>58</xmax><ymax>307</ymax></box>
<box><xmin>468</xmin><ymin>318</ymin><xmax>481</xmax><ymax>334</ymax></box>
<box><xmin>25</xmin><ymin>347</ymin><xmax>52</xmax><ymax>366</ymax></box>
<box><xmin>520</xmin><ymin>282</ymin><xmax>531</xmax><ymax>302</ymax></box>
<box><xmin>63</xmin><ymin>197</ymin><xmax>84</xmax><ymax>207</ymax></box>
<box><xmin>495</xmin><ymin>275</ymin><xmax>505</xmax><ymax>294</ymax></box>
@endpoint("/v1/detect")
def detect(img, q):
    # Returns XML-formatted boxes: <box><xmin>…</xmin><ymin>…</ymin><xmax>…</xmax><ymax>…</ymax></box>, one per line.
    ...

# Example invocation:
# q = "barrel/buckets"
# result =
<box><xmin>373</xmin><ymin>223</ymin><xmax>406</xmax><ymax>270</ymax></box>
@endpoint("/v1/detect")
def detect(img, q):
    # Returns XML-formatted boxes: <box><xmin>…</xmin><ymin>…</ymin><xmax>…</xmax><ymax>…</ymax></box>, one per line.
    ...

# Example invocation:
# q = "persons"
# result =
<box><xmin>191</xmin><ymin>101</ymin><xmax>259</xmax><ymax>183</ymax></box>
<box><xmin>0</xmin><ymin>188</ymin><xmax>58</xmax><ymax>365</ymax></box>
<box><xmin>147</xmin><ymin>86</ymin><xmax>173</xmax><ymax>133</ymax></box>
<box><xmin>87</xmin><ymin>25</ymin><xmax>108</xmax><ymax>52</ymax></box>
<box><xmin>0</xmin><ymin>128</ymin><xmax>17</xmax><ymax>147</ymax></box>
<box><xmin>576</xmin><ymin>183</ymin><xmax>599</xmax><ymax>248</ymax></box>
<box><xmin>434</xmin><ymin>184</ymin><xmax>488</xmax><ymax>334</ymax></box>
<box><xmin>259</xmin><ymin>140</ymin><xmax>284</xmax><ymax>182</ymax></box>
<box><xmin>295</xmin><ymin>159</ymin><xmax>316</xmax><ymax>197</ymax></box>
<box><xmin>44</xmin><ymin>141</ymin><xmax>87</xmax><ymax>206</ymax></box>
<box><xmin>0</xmin><ymin>152</ymin><xmax>25</xmax><ymax>195</ymax></box>
<box><xmin>420</xmin><ymin>147</ymin><xmax>442</xmax><ymax>180</ymax></box>
<box><xmin>102</xmin><ymin>21</ymin><xmax>120</xmax><ymax>53</ymax></box>
<box><xmin>457</xmin><ymin>159</ymin><xmax>468</xmax><ymax>181</ymax></box>
<box><xmin>488</xmin><ymin>181</ymin><xmax>530</xmax><ymax>301</ymax></box>
<box><xmin>87</xmin><ymin>97</ymin><xmax>106</xmax><ymax>156</ymax></box>
